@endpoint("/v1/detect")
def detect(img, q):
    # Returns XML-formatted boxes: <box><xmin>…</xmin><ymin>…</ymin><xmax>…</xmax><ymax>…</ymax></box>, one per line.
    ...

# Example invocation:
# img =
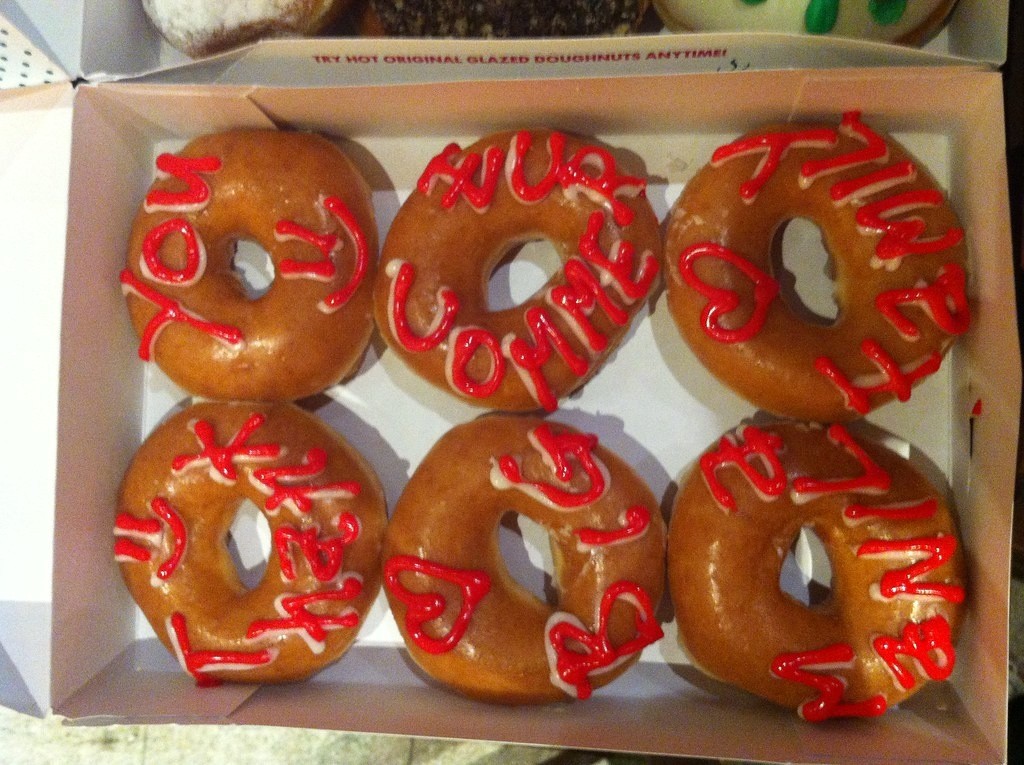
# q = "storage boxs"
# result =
<box><xmin>1</xmin><ymin>66</ymin><xmax>1022</xmax><ymax>765</ymax></box>
<box><xmin>0</xmin><ymin>0</ymin><xmax>1012</xmax><ymax>83</ymax></box>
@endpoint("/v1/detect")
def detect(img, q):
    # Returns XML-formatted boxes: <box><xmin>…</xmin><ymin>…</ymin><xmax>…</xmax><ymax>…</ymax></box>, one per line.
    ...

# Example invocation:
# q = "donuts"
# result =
<box><xmin>660</xmin><ymin>110</ymin><xmax>973</xmax><ymax>425</ymax></box>
<box><xmin>137</xmin><ymin>0</ymin><xmax>963</xmax><ymax>64</ymax></box>
<box><xmin>372</xmin><ymin>130</ymin><xmax>662</xmax><ymax>417</ymax></box>
<box><xmin>119</xmin><ymin>129</ymin><xmax>380</xmax><ymax>405</ymax></box>
<box><xmin>382</xmin><ymin>413</ymin><xmax>667</xmax><ymax>707</ymax></box>
<box><xmin>113</xmin><ymin>396</ymin><xmax>392</xmax><ymax>687</ymax></box>
<box><xmin>662</xmin><ymin>415</ymin><xmax>968</xmax><ymax>721</ymax></box>
